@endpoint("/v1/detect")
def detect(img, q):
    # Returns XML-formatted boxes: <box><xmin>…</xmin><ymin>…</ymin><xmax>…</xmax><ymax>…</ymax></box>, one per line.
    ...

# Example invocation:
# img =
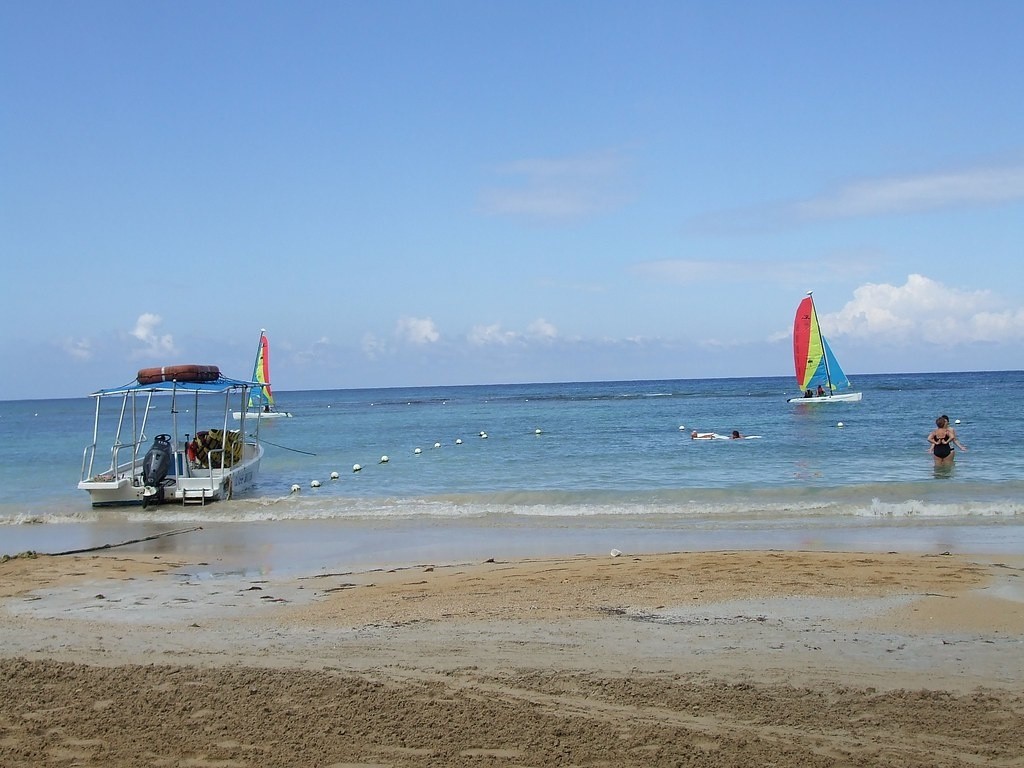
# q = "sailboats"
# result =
<box><xmin>233</xmin><ymin>328</ymin><xmax>293</xmax><ymax>420</ymax></box>
<box><xmin>787</xmin><ymin>291</ymin><xmax>863</xmax><ymax>403</ymax></box>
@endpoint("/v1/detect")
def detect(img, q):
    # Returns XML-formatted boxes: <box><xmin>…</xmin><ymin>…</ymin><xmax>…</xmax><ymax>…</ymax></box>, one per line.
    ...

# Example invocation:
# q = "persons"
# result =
<box><xmin>817</xmin><ymin>385</ymin><xmax>825</xmax><ymax>397</ymax></box>
<box><xmin>927</xmin><ymin>415</ymin><xmax>967</xmax><ymax>466</ymax></box>
<box><xmin>727</xmin><ymin>430</ymin><xmax>741</xmax><ymax>439</ymax></box>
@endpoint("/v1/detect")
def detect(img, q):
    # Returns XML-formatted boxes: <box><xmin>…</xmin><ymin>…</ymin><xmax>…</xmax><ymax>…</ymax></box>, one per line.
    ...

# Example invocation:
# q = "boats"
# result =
<box><xmin>77</xmin><ymin>365</ymin><xmax>264</xmax><ymax>509</ymax></box>
